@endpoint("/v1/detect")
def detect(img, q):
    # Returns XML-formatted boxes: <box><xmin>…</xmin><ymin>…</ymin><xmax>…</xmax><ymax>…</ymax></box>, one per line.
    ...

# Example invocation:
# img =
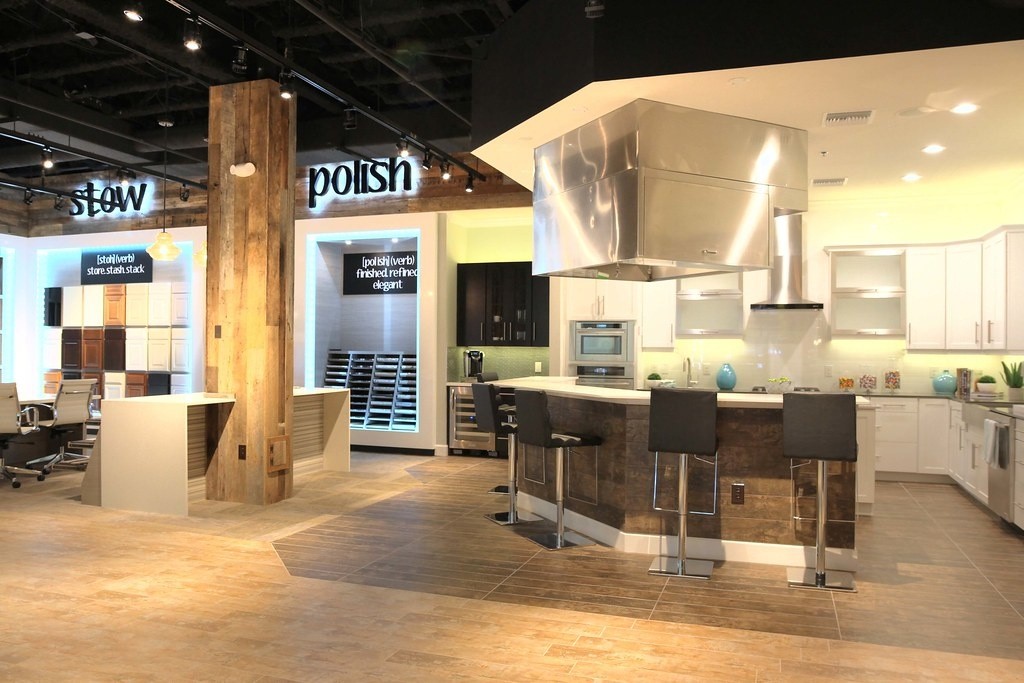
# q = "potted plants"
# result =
<box><xmin>1000</xmin><ymin>361</ymin><xmax>1023</xmax><ymax>403</ymax></box>
<box><xmin>977</xmin><ymin>375</ymin><xmax>996</xmax><ymax>393</ymax></box>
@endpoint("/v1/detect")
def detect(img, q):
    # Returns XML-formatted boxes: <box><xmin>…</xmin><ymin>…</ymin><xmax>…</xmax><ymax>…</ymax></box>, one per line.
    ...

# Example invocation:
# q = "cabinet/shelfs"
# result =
<box><xmin>824</xmin><ymin>223</ymin><xmax>1024</xmax><ymax>352</ymax></box>
<box><xmin>456</xmin><ymin>262</ymin><xmax>548</xmax><ymax>349</ymax></box>
<box><xmin>868</xmin><ymin>396</ymin><xmax>1024</xmax><ymax>536</ymax></box>
<box><xmin>575</xmin><ymin>278</ymin><xmax>675</xmax><ymax>350</ymax></box>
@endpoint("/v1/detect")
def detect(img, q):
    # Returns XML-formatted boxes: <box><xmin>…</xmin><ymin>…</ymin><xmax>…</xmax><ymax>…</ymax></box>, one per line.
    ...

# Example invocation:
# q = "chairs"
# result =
<box><xmin>0</xmin><ymin>382</ymin><xmax>46</xmax><ymax>488</ymax></box>
<box><xmin>23</xmin><ymin>377</ymin><xmax>99</xmax><ymax>474</ymax></box>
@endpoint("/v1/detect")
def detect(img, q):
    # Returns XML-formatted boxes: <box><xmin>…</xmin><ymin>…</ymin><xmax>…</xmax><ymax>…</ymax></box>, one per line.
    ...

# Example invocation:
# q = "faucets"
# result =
<box><xmin>682</xmin><ymin>357</ymin><xmax>700</xmax><ymax>388</ymax></box>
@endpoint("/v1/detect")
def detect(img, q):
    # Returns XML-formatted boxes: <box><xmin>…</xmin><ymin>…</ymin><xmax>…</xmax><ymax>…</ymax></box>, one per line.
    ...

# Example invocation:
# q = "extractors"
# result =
<box><xmin>750</xmin><ymin>212</ymin><xmax>825</xmax><ymax>313</ymax></box>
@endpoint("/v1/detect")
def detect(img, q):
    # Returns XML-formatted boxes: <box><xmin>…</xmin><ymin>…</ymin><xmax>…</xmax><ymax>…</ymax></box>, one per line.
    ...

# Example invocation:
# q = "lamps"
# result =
<box><xmin>397</xmin><ymin>135</ymin><xmax>409</xmax><ymax>157</ymax></box>
<box><xmin>423</xmin><ymin>148</ymin><xmax>434</xmax><ymax>170</ymax></box>
<box><xmin>146</xmin><ymin>115</ymin><xmax>182</xmax><ymax>260</ymax></box>
<box><xmin>124</xmin><ymin>3</ymin><xmax>146</xmax><ymax>22</ymax></box>
<box><xmin>184</xmin><ymin>14</ymin><xmax>202</xmax><ymax>50</ymax></box>
<box><xmin>465</xmin><ymin>173</ymin><xmax>474</xmax><ymax>193</ymax></box>
<box><xmin>343</xmin><ymin>102</ymin><xmax>357</xmax><ymax>130</ymax></box>
<box><xmin>40</xmin><ymin>145</ymin><xmax>54</xmax><ymax>168</ymax></box>
<box><xmin>440</xmin><ymin>157</ymin><xmax>454</xmax><ymax>179</ymax></box>
<box><xmin>231</xmin><ymin>41</ymin><xmax>249</xmax><ymax>75</ymax></box>
<box><xmin>278</xmin><ymin>48</ymin><xmax>297</xmax><ymax>99</ymax></box>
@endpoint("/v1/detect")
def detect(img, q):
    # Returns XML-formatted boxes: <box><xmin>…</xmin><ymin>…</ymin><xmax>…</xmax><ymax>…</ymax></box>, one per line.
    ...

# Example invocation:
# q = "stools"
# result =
<box><xmin>648</xmin><ymin>388</ymin><xmax>719</xmax><ymax>578</ymax></box>
<box><xmin>472</xmin><ymin>383</ymin><xmax>528</xmax><ymax>526</ymax></box>
<box><xmin>513</xmin><ymin>387</ymin><xmax>602</xmax><ymax>555</ymax></box>
<box><xmin>782</xmin><ymin>392</ymin><xmax>855</xmax><ymax>592</ymax></box>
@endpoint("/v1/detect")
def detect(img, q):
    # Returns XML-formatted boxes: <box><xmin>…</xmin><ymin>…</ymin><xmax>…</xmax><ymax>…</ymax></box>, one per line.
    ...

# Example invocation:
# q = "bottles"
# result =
<box><xmin>717</xmin><ymin>363</ymin><xmax>736</xmax><ymax>390</ymax></box>
<box><xmin>931</xmin><ymin>370</ymin><xmax>958</xmax><ymax>396</ymax></box>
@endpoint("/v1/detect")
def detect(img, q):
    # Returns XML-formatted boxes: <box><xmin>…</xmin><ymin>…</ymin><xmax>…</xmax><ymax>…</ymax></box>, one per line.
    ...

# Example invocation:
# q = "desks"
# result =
<box><xmin>294</xmin><ymin>386</ymin><xmax>351</xmax><ymax>472</ymax></box>
<box><xmin>19</xmin><ymin>394</ymin><xmax>102</xmax><ymax>461</ymax></box>
<box><xmin>101</xmin><ymin>393</ymin><xmax>237</xmax><ymax>517</ymax></box>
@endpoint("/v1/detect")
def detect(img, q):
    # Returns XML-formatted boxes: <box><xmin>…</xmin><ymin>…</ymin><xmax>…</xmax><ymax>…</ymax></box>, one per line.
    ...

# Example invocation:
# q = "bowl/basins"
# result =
<box><xmin>645</xmin><ymin>379</ymin><xmax>674</xmax><ymax>388</ymax></box>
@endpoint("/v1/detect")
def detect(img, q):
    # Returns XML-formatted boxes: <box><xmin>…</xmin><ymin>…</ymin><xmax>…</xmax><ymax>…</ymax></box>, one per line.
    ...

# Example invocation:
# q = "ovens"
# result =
<box><xmin>569</xmin><ymin>320</ymin><xmax>635</xmax><ymax>365</ymax></box>
<box><xmin>986</xmin><ymin>410</ymin><xmax>1016</xmax><ymax>524</ymax></box>
